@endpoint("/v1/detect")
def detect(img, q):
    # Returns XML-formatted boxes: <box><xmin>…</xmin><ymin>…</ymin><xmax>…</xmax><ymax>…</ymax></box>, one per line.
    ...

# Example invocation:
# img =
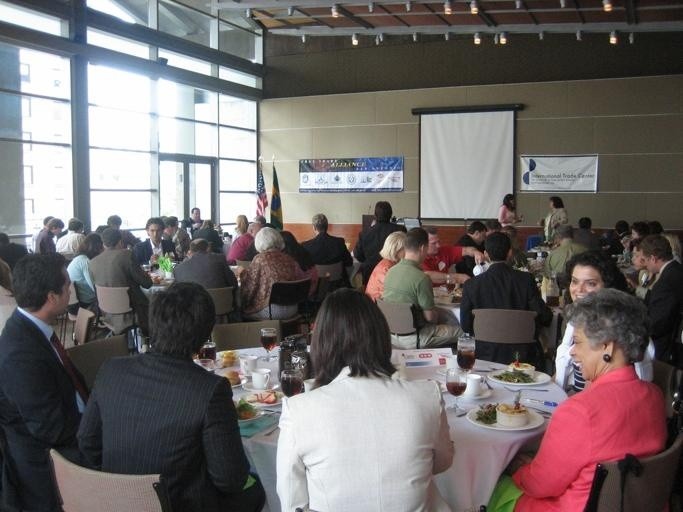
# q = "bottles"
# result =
<box><xmin>513</xmin><ymin>389</ymin><xmax>521</xmax><ymax>404</ymax></box>
<box><xmin>143</xmin><ymin>336</ymin><xmax>151</xmax><ymax>353</ymax></box>
<box><xmin>540</xmin><ymin>270</ymin><xmax>560</xmax><ymax>305</ymax></box>
<box><xmin>278</xmin><ymin>333</ymin><xmax>312</xmax><ymax>382</ymax></box>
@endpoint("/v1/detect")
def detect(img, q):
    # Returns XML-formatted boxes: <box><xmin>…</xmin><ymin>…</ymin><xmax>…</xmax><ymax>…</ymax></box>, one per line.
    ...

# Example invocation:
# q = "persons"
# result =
<box><xmin>74</xmin><ymin>278</ymin><xmax>264</xmax><ymax>510</ymax></box>
<box><xmin>0</xmin><ymin>247</ymin><xmax>102</xmax><ymax>449</ymax></box>
<box><xmin>276</xmin><ymin>286</ymin><xmax>459</xmax><ymax>512</ymax></box>
<box><xmin>0</xmin><ymin>194</ymin><xmax>681</xmax><ymax>371</ymax></box>
<box><xmin>486</xmin><ymin>286</ymin><xmax>670</xmax><ymax>512</ymax></box>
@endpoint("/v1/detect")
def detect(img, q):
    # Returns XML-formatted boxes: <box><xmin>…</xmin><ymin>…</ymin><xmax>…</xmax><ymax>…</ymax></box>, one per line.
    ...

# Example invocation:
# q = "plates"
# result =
<box><xmin>466</xmin><ymin>406</ymin><xmax>544</xmax><ymax>431</ymax></box>
<box><xmin>245</xmin><ymin>393</ymin><xmax>282</xmax><ymax>406</ymax></box>
<box><xmin>487</xmin><ymin>369</ymin><xmax>550</xmax><ymax>384</ymax></box>
<box><xmin>237</xmin><ymin>408</ymin><xmax>263</xmax><ymax>422</ymax></box>
<box><xmin>243</xmin><ymin>382</ymin><xmax>279</xmax><ymax>391</ymax></box>
<box><xmin>459</xmin><ymin>388</ymin><xmax>491</xmax><ymax>400</ymax></box>
<box><xmin>230</xmin><ymin>375</ymin><xmax>247</xmax><ymax>388</ymax></box>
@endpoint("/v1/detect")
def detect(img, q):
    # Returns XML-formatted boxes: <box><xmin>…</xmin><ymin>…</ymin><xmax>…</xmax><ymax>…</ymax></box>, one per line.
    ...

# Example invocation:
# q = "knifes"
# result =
<box><xmin>527</xmin><ymin>398</ymin><xmax>559</xmax><ymax>406</ymax></box>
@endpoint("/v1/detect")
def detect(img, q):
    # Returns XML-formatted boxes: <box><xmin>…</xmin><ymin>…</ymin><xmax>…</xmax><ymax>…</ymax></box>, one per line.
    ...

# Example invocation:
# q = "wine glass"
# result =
<box><xmin>260</xmin><ymin>328</ymin><xmax>277</xmax><ymax>360</ymax></box>
<box><xmin>445</xmin><ymin>367</ymin><xmax>466</xmax><ymax>412</ymax></box>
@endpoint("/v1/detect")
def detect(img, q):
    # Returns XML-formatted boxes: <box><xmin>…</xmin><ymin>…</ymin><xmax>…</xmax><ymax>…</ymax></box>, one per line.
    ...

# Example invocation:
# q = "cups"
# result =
<box><xmin>456</xmin><ymin>336</ymin><xmax>476</xmax><ymax>371</ymax></box>
<box><xmin>446</xmin><ymin>273</ymin><xmax>455</xmax><ymax>293</ymax></box>
<box><xmin>197</xmin><ymin>342</ymin><xmax>215</xmax><ymax>373</ymax></box>
<box><xmin>251</xmin><ymin>368</ymin><xmax>271</xmax><ymax>389</ymax></box>
<box><xmin>238</xmin><ymin>356</ymin><xmax>257</xmax><ymax>375</ymax></box>
<box><xmin>280</xmin><ymin>370</ymin><xmax>304</xmax><ymax>397</ymax></box>
<box><xmin>142</xmin><ymin>261</ymin><xmax>173</xmax><ymax>280</ymax></box>
<box><xmin>466</xmin><ymin>374</ymin><xmax>485</xmax><ymax>396</ymax></box>
<box><xmin>472</xmin><ymin>264</ymin><xmax>483</xmax><ymax>277</ymax></box>
<box><xmin>302</xmin><ymin>378</ymin><xmax>317</xmax><ymax>392</ymax></box>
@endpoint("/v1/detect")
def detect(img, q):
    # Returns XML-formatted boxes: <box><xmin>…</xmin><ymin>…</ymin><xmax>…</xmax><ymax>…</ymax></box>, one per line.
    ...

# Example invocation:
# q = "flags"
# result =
<box><xmin>256</xmin><ymin>158</ymin><xmax>268</xmax><ymax>221</ymax></box>
<box><xmin>268</xmin><ymin>162</ymin><xmax>283</xmax><ymax>232</ymax></box>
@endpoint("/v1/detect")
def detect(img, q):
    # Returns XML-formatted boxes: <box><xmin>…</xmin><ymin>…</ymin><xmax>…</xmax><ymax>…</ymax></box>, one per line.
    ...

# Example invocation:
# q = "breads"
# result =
<box><xmin>224</xmin><ymin>371</ymin><xmax>240</xmax><ymax>385</ymax></box>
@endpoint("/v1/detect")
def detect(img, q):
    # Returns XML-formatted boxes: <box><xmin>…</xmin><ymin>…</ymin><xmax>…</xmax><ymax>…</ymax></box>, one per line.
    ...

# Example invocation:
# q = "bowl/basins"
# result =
<box><xmin>495</xmin><ymin>404</ymin><xmax>528</xmax><ymax>426</ymax></box>
<box><xmin>509</xmin><ymin>362</ymin><xmax>534</xmax><ymax>379</ymax></box>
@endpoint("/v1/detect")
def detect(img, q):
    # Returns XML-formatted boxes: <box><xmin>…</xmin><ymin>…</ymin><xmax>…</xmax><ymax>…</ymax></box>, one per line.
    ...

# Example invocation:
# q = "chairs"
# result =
<box><xmin>66</xmin><ymin>334</ymin><xmax>130</xmax><ymax>383</ymax></box>
<box><xmin>43</xmin><ymin>446</ymin><xmax>171</xmax><ymax>512</ymax></box>
<box><xmin>211</xmin><ymin>320</ymin><xmax>280</xmax><ymax>353</ymax></box>
<box><xmin>74</xmin><ymin>307</ymin><xmax>96</xmax><ymax>345</ymax></box>
<box><xmin>315</xmin><ymin>261</ymin><xmax>343</xmax><ymax>281</ymax></box>
<box><xmin>432</xmin><ymin>286</ymin><xmax>563</xmax><ymax>352</ymax></box>
<box><xmin>268</xmin><ymin>277</ymin><xmax>313</xmax><ymax>332</ymax></box>
<box><xmin>312</xmin><ymin>272</ymin><xmax>331</xmax><ymax>303</ymax></box>
<box><xmin>470</xmin><ymin>307</ymin><xmax>540</xmax><ymax>346</ymax></box>
<box><xmin>235</xmin><ymin>258</ymin><xmax>253</xmax><ymax>271</ymax></box>
<box><xmin>583</xmin><ymin>393</ymin><xmax>683</xmax><ymax>512</ymax></box>
<box><xmin>94</xmin><ymin>282</ymin><xmax>140</xmax><ymax>354</ymax></box>
<box><xmin>207</xmin><ymin>287</ymin><xmax>236</xmax><ymax>316</ymax></box>
<box><xmin>651</xmin><ymin>353</ymin><xmax>677</xmax><ymax>420</ymax></box>
<box><xmin>374</xmin><ymin>296</ymin><xmax>419</xmax><ymax>348</ymax></box>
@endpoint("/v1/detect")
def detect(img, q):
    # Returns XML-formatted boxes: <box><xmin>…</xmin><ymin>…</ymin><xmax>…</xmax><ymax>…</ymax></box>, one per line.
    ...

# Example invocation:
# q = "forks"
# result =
<box><xmin>504</xmin><ymin>385</ymin><xmax>550</xmax><ymax>392</ymax></box>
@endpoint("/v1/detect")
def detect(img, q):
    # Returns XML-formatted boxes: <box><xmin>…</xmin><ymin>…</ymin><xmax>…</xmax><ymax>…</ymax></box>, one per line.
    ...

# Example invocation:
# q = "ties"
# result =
<box><xmin>51</xmin><ymin>332</ymin><xmax>89</xmax><ymax>405</ymax></box>
